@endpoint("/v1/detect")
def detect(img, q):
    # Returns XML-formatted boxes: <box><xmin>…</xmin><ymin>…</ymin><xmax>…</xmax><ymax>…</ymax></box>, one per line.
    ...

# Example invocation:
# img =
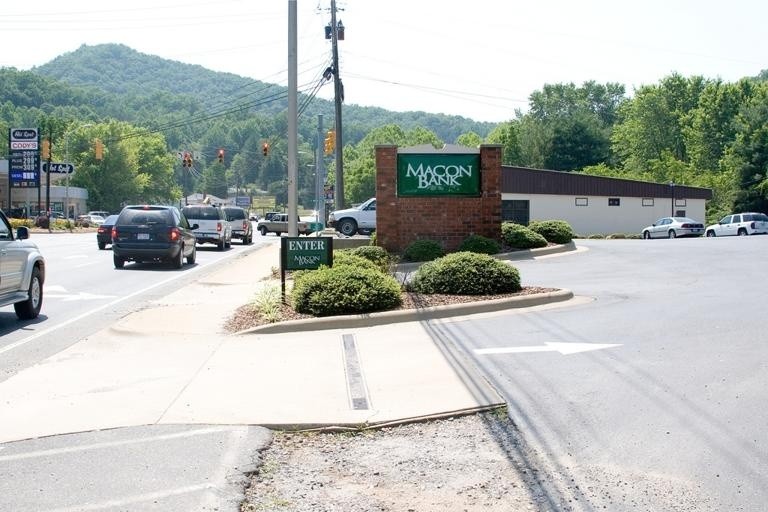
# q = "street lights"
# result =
<box><xmin>670</xmin><ymin>180</ymin><xmax>675</xmax><ymax>216</ymax></box>
<box><xmin>66</xmin><ymin>124</ymin><xmax>94</xmax><ymax>219</ymax></box>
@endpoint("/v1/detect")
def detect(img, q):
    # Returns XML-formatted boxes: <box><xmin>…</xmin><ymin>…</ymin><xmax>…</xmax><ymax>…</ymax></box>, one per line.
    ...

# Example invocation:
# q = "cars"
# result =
<box><xmin>328</xmin><ymin>197</ymin><xmax>377</xmax><ymax>236</ymax></box>
<box><xmin>705</xmin><ymin>212</ymin><xmax>768</xmax><ymax>237</ymax></box>
<box><xmin>641</xmin><ymin>217</ymin><xmax>704</xmax><ymax>239</ymax></box>
<box><xmin>265</xmin><ymin>213</ymin><xmax>280</xmax><ymax>220</ymax></box>
<box><xmin>0</xmin><ymin>204</ymin><xmax>64</xmax><ymax>321</ymax></box>
<box><xmin>77</xmin><ymin>204</ymin><xmax>258</xmax><ymax>268</ymax></box>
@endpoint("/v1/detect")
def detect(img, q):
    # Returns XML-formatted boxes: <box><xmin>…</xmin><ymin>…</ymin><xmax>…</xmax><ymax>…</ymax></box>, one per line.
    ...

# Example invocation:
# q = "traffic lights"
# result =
<box><xmin>263</xmin><ymin>142</ymin><xmax>269</xmax><ymax>157</ymax></box>
<box><xmin>218</xmin><ymin>148</ymin><xmax>225</xmax><ymax>163</ymax></box>
<box><xmin>182</xmin><ymin>152</ymin><xmax>192</xmax><ymax>169</ymax></box>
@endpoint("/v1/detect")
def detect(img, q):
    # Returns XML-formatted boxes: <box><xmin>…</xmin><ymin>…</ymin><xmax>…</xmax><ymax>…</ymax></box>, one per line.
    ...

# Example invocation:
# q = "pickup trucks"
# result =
<box><xmin>257</xmin><ymin>214</ymin><xmax>321</xmax><ymax>235</ymax></box>
